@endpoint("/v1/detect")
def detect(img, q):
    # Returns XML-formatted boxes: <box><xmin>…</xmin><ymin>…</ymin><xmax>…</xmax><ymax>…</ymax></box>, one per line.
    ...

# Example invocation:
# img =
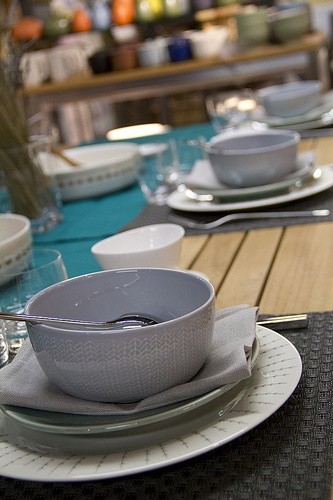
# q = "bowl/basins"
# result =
<box><xmin>186</xmin><ymin>25</ymin><xmax>228</xmax><ymax>58</ymax></box>
<box><xmin>0</xmin><ymin>212</ymin><xmax>33</xmax><ymax>287</ymax></box>
<box><xmin>265</xmin><ymin>5</ymin><xmax>310</xmax><ymax>43</ymax></box>
<box><xmin>205</xmin><ymin>127</ymin><xmax>302</xmax><ymax>188</ymax></box>
<box><xmin>262</xmin><ymin>81</ymin><xmax>321</xmax><ymax>117</ymax></box>
<box><xmin>34</xmin><ymin>143</ymin><xmax>145</xmax><ymax>202</ymax></box>
<box><xmin>23</xmin><ymin>266</ymin><xmax>216</xmax><ymax>405</ymax></box>
<box><xmin>90</xmin><ymin>222</ymin><xmax>185</xmax><ymax>271</ymax></box>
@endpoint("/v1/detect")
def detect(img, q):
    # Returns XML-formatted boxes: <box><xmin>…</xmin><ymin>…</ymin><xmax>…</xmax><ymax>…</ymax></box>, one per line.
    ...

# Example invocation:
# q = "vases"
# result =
<box><xmin>0</xmin><ymin>137</ymin><xmax>62</xmax><ymax>234</ymax></box>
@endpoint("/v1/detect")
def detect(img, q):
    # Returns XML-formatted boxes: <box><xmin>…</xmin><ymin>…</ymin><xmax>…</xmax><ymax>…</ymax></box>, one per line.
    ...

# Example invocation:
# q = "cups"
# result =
<box><xmin>126</xmin><ymin>138</ymin><xmax>184</xmax><ymax>208</ymax></box>
<box><xmin>11</xmin><ymin>0</ymin><xmax>192</xmax><ymax>42</ymax></box>
<box><xmin>0</xmin><ymin>247</ymin><xmax>69</xmax><ymax>367</ymax></box>
<box><xmin>204</xmin><ymin>90</ymin><xmax>249</xmax><ymax>134</ymax></box>
<box><xmin>18</xmin><ymin>25</ymin><xmax>193</xmax><ymax>87</ymax></box>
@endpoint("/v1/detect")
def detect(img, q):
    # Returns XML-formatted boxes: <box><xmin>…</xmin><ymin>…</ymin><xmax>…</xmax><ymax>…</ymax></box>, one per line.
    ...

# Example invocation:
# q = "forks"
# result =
<box><xmin>167</xmin><ymin>205</ymin><xmax>332</xmax><ymax>229</ymax></box>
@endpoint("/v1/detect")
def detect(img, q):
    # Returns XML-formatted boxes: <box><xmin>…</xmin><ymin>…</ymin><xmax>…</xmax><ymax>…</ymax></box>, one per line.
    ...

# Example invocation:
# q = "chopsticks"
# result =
<box><xmin>257</xmin><ymin>313</ymin><xmax>309</xmax><ymax>330</ymax></box>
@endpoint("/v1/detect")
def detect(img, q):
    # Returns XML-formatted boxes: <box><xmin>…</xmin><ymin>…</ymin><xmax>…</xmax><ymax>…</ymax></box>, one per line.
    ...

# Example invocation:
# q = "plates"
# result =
<box><xmin>106</xmin><ymin>123</ymin><xmax>168</xmax><ymax>142</ymax></box>
<box><xmin>166</xmin><ymin>162</ymin><xmax>333</xmax><ymax>212</ymax></box>
<box><xmin>0</xmin><ymin>323</ymin><xmax>303</xmax><ymax>482</ymax></box>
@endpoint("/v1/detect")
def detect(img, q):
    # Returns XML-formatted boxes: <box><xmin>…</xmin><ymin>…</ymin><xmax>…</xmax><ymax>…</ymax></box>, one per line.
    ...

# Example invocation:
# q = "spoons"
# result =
<box><xmin>0</xmin><ymin>311</ymin><xmax>160</xmax><ymax>329</ymax></box>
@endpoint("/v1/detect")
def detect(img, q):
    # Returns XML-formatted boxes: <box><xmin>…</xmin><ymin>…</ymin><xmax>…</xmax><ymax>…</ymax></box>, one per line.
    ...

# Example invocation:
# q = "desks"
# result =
<box><xmin>23</xmin><ymin>29</ymin><xmax>325</xmax><ymax>146</ymax></box>
<box><xmin>0</xmin><ymin>106</ymin><xmax>333</xmax><ymax>500</ymax></box>
